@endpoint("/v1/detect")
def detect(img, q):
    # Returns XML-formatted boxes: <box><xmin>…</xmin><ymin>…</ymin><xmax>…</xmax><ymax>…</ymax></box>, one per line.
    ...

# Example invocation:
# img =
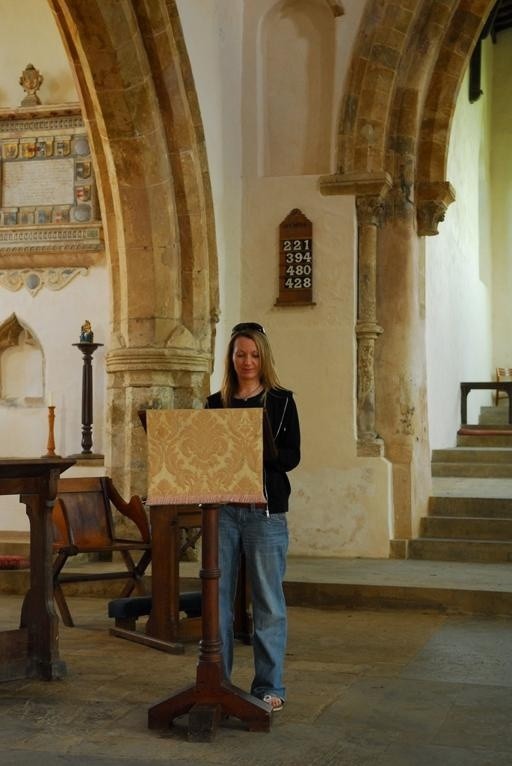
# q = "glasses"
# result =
<box><xmin>232</xmin><ymin>323</ymin><xmax>267</xmax><ymax>335</ymax></box>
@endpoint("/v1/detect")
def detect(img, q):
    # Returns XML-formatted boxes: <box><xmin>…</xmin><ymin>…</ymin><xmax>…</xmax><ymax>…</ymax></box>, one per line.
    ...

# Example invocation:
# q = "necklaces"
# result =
<box><xmin>232</xmin><ymin>383</ymin><xmax>264</xmax><ymax>402</ymax></box>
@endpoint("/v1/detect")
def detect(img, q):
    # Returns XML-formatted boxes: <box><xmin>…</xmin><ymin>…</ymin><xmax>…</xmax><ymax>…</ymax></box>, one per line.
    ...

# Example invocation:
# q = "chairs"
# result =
<box><xmin>495</xmin><ymin>367</ymin><xmax>512</xmax><ymax>408</ymax></box>
<box><xmin>53</xmin><ymin>476</ymin><xmax>157</xmax><ymax>630</ymax></box>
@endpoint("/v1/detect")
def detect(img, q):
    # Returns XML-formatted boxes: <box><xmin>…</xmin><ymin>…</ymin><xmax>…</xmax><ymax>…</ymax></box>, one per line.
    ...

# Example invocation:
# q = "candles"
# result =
<box><xmin>49</xmin><ymin>391</ymin><xmax>55</xmax><ymax>406</ymax></box>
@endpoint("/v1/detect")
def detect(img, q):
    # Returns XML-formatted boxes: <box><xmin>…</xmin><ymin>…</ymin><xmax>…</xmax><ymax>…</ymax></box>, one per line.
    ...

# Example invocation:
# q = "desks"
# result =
<box><xmin>0</xmin><ymin>459</ymin><xmax>76</xmax><ymax>685</ymax></box>
<box><xmin>459</xmin><ymin>381</ymin><xmax>512</xmax><ymax>426</ymax></box>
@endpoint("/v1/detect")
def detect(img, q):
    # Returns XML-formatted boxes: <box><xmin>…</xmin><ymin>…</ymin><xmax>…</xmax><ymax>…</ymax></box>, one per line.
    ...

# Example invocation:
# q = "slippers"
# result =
<box><xmin>261</xmin><ymin>696</ymin><xmax>284</xmax><ymax>711</ymax></box>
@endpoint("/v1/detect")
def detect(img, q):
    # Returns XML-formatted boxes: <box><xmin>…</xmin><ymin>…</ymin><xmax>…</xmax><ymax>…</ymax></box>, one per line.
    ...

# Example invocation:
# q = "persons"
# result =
<box><xmin>200</xmin><ymin>318</ymin><xmax>301</xmax><ymax>711</ymax></box>
